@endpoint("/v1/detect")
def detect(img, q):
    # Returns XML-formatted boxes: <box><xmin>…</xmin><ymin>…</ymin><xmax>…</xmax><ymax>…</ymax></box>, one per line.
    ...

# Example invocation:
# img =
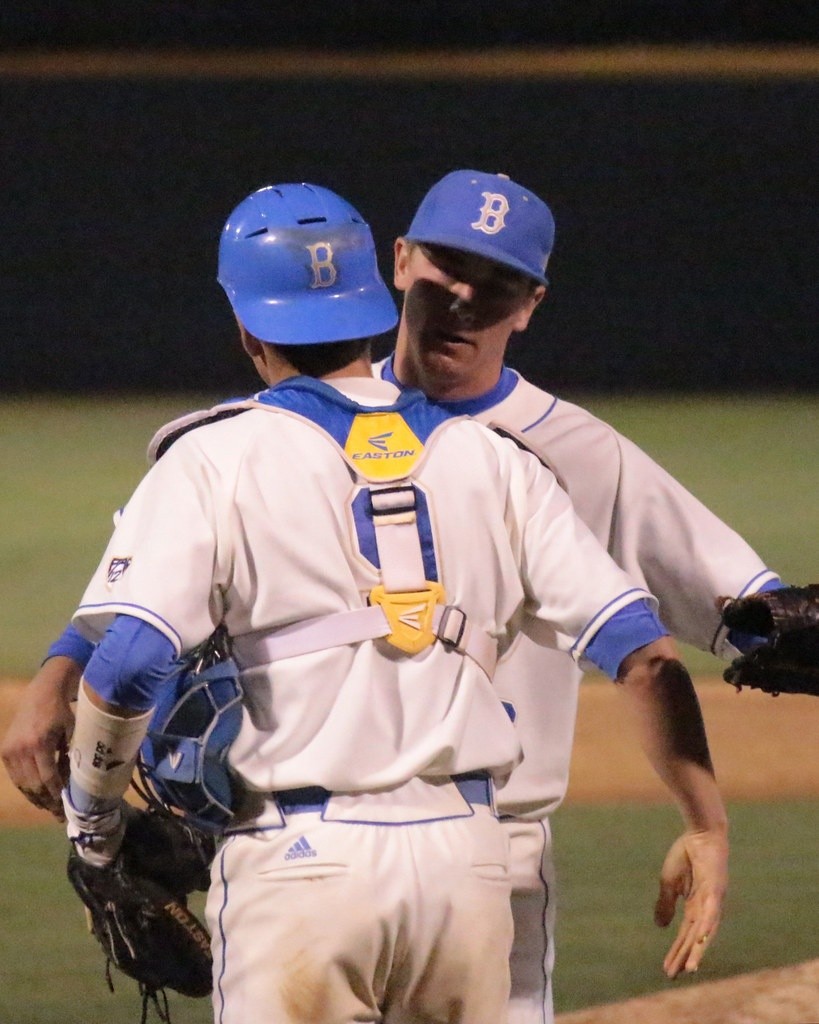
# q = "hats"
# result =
<box><xmin>406</xmin><ymin>166</ymin><xmax>556</xmax><ymax>292</ymax></box>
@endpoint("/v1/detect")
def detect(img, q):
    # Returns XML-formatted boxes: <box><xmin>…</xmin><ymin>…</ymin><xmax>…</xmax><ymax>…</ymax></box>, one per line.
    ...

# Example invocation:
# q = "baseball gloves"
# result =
<box><xmin>63</xmin><ymin>810</ymin><xmax>215</xmax><ymax>999</ymax></box>
<box><xmin>720</xmin><ymin>578</ymin><xmax>819</xmax><ymax>701</ymax></box>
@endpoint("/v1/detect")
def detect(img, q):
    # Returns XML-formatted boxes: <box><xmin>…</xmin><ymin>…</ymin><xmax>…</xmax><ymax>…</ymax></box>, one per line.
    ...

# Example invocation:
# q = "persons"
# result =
<box><xmin>60</xmin><ymin>181</ymin><xmax>729</xmax><ymax>1024</ymax></box>
<box><xmin>0</xmin><ymin>167</ymin><xmax>799</xmax><ymax>1024</ymax></box>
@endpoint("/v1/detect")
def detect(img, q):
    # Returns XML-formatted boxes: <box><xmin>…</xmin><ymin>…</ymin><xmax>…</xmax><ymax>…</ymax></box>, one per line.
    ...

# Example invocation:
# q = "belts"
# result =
<box><xmin>277</xmin><ymin>766</ymin><xmax>490</xmax><ymax>815</ymax></box>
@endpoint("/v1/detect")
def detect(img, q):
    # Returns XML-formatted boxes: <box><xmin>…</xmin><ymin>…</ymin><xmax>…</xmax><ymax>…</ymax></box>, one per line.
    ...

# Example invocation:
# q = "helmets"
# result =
<box><xmin>217</xmin><ymin>183</ymin><xmax>400</xmax><ymax>346</ymax></box>
